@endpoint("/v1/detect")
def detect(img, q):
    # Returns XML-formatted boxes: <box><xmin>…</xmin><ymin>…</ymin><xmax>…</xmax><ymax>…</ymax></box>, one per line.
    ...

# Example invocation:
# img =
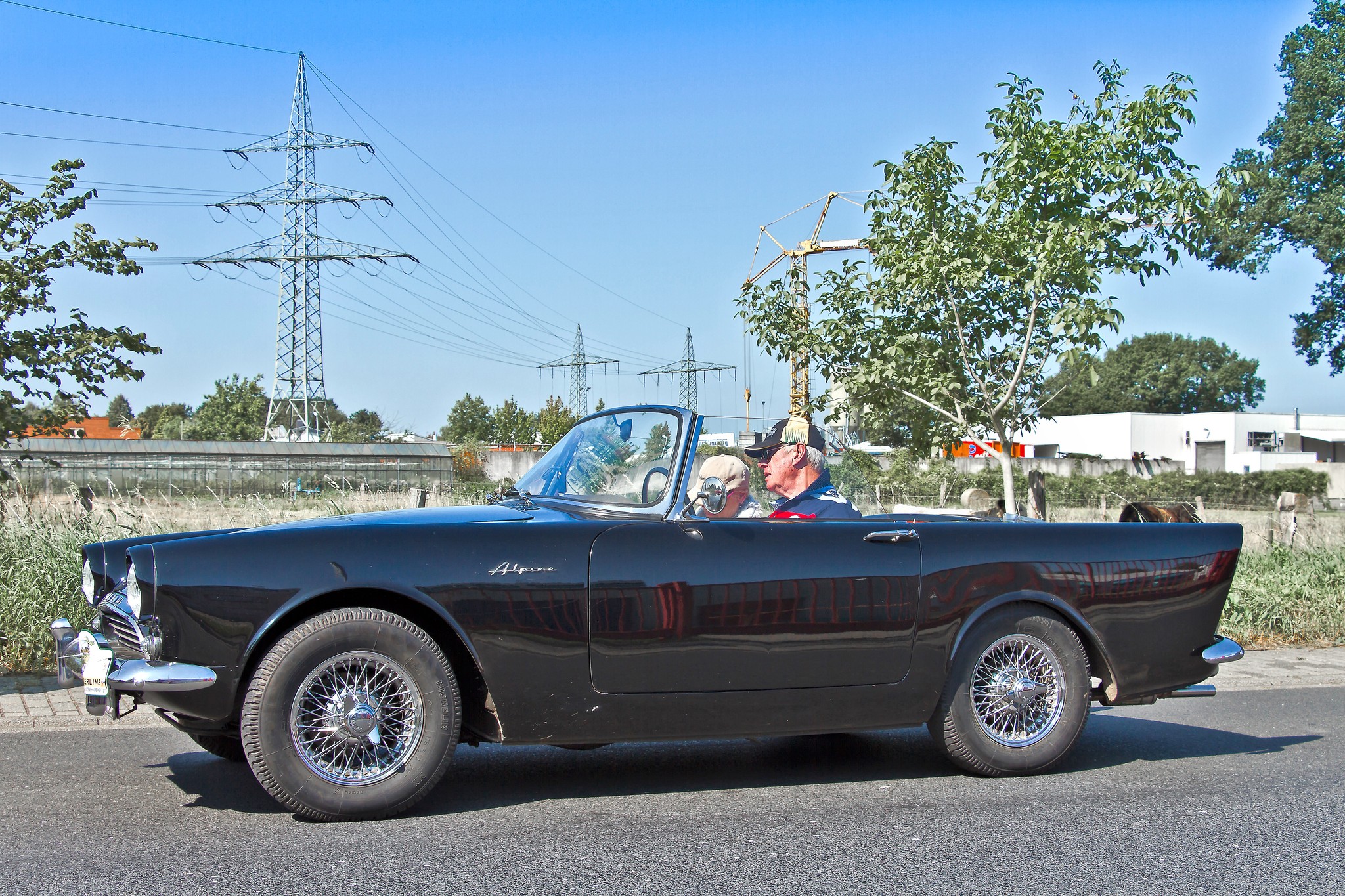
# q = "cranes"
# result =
<box><xmin>737</xmin><ymin>192</ymin><xmax>899</xmax><ymax>451</ymax></box>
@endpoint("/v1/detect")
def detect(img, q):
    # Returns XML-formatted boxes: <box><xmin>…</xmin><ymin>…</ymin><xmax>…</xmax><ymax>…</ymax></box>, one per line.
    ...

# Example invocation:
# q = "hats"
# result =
<box><xmin>683</xmin><ymin>452</ymin><xmax>750</xmax><ymax>506</ymax></box>
<box><xmin>743</xmin><ymin>415</ymin><xmax>825</xmax><ymax>458</ymax></box>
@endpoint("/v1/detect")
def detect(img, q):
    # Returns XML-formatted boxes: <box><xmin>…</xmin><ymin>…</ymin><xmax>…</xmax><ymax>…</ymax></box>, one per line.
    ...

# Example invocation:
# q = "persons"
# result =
<box><xmin>678</xmin><ymin>453</ymin><xmax>768</xmax><ymax>518</ymax></box>
<box><xmin>744</xmin><ymin>414</ymin><xmax>864</xmax><ymax>520</ymax></box>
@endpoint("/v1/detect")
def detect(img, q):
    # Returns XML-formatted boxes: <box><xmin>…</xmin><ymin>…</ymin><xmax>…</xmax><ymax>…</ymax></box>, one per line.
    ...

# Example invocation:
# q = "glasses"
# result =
<box><xmin>759</xmin><ymin>443</ymin><xmax>797</xmax><ymax>461</ymax></box>
<box><xmin>693</xmin><ymin>488</ymin><xmax>735</xmax><ymax>509</ymax></box>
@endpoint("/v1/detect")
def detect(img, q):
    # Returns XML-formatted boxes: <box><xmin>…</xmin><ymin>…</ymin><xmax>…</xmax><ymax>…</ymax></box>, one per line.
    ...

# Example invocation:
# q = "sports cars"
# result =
<box><xmin>52</xmin><ymin>405</ymin><xmax>1246</xmax><ymax>822</ymax></box>
